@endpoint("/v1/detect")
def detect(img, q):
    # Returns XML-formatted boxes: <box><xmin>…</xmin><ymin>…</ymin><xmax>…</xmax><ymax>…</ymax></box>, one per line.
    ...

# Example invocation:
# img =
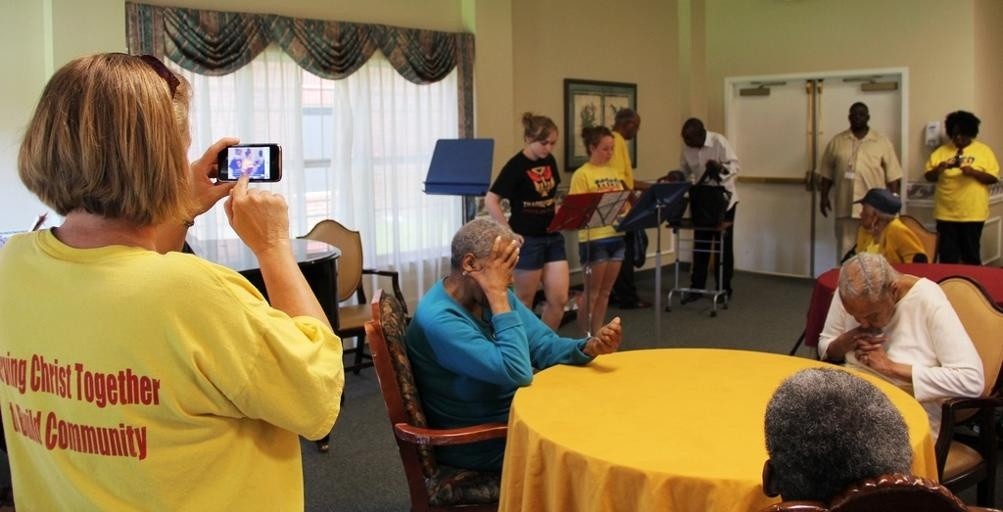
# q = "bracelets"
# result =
<box><xmin>166</xmin><ymin>212</ymin><xmax>194</xmax><ymax>227</ymax></box>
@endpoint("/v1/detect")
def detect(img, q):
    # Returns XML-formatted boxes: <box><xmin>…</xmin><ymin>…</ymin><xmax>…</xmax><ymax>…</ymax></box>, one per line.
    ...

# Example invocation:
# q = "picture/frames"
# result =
<box><xmin>562</xmin><ymin>74</ymin><xmax>638</xmax><ymax>172</ymax></box>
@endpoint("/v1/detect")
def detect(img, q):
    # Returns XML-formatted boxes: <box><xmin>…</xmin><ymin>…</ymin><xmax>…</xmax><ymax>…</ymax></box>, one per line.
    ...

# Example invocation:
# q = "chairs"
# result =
<box><xmin>760</xmin><ymin>476</ymin><xmax>1002</xmax><ymax>512</ymax></box>
<box><xmin>363</xmin><ymin>286</ymin><xmax>509</xmax><ymax>512</ymax></box>
<box><xmin>296</xmin><ymin>218</ymin><xmax>407</xmax><ymax>375</ymax></box>
<box><xmin>929</xmin><ymin>275</ymin><xmax>1003</xmax><ymax>512</ymax></box>
<box><xmin>855</xmin><ymin>215</ymin><xmax>939</xmax><ymax>262</ymax></box>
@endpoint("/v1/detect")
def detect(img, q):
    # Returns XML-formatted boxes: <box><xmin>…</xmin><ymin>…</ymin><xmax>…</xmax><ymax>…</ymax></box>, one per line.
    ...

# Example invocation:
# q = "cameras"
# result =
<box><xmin>952</xmin><ymin>155</ymin><xmax>961</xmax><ymax>168</ymax></box>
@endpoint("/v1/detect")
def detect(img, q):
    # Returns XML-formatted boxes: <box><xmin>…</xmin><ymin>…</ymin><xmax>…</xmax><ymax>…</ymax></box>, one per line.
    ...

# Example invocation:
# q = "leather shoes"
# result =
<box><xmin>637</xmin><ymin>300</ymin><xmax>651</xmax><ymax>308</ymax></box>
<box><xmin>687</xmin><ymin>292</ymin><xmax>703</xmax><ymax>302</ymax></box>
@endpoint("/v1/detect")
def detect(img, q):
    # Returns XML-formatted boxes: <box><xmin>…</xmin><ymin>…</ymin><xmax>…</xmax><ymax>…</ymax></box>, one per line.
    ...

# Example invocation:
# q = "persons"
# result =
<box><xmin>609</xmin><ymin>108</ymin><xmax>653</xmax><ymax>309</ymax></box>
<box><xmin>818</xmin><ymin>101</ymin><xmax>901</xmax><ymax>263</ymax></box>
<box><xmin>243</xmin><ymin>149</ymin><xmax>254</xmax><ymax>176</ymax></box>
<box><xmin>406</xmin><ymin>216</ymin><xmax>620</xmax><ymax>468</ymax></box>
<box><xmin>484</xmin><ymin>112</ymin><xmax>569</xmax><ymax>334</ymax></box>
<box><xmin>679</xmin><ymin>118</ymin><xmax>738</xmax><ymax>303</ymax></box>
<box><xmin>254</xmin><ymin>149</ymin><xmax>264</xmax><ymax>177</ymax></box>
<box><xmin>229</xmin><ymin>149</ymin><xmax>242</xmax><ymax>177</ymax></box>
<box><xmin>762</xmin><ymin>367</ymin><xmax>911</xmax><ymax>504</ymax></box>
<box><xmin>569</xmin><ymin>124</ymin><xmax>630</xmax><ymax>337</ymax></box>
<box><xmin>923</xmin><ymin>111</ymin><xmax>1000</xmax><ymax>264</ymax></box>
<box><xmin>816</xmin><ymin>251</ymin><xmax>985</xmax><ymax>445</ymax></box>
<box><xmin>853</xmin><ymin>188</ymin><xmax>931</xmax><ymax>264</ymax></box>
<box><xmin>0</xmin><ymin>51</ymin><xmax>344</xmax><ymax>510</ymax></box>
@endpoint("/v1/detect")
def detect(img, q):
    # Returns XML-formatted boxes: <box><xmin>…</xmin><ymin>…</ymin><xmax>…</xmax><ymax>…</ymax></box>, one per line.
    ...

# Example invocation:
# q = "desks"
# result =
<box><xmin>665</xmin><ymin>218</ymin><xmax>733</xmax><ymax>316</ymax></box>
<box><xmin>499</xmin><ymin>346</ymin><xmax>946</xmax><ymax>512</ymax></box>
<box><xmin>809</xmin><ymin>262</ymin><xmax>1003</xmax><ymax>349</ymax></box>
<box><xmin>186</xmin><ymin>228</ymin><xmax>343</xmax><ymax>454</ymax></box>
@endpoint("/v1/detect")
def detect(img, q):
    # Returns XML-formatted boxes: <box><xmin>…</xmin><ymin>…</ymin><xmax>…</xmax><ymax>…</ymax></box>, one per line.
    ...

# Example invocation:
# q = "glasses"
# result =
<box><xmin>109</xmin><ymin>52</ymin><xmax>180</xmax><ymax>99</ymax></box>
<box><xmin>630</xmin><ymin>121</ymin><xmax>640</xmax><ymax>130</ymax></box>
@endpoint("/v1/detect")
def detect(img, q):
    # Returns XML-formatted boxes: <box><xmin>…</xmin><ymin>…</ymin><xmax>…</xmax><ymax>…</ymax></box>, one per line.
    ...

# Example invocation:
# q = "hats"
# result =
<box><xmin>849</xmin><ymin>188</ymin><xmax>902</xmax><ymax>215</ymax></box>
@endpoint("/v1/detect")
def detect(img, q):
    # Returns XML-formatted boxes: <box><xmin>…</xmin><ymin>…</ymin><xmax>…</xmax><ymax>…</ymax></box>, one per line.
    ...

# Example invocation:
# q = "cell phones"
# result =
<box><xmin>217</xmin><ymin>143</ymin><xmax>283</xmax><ymax>182</ymax></box>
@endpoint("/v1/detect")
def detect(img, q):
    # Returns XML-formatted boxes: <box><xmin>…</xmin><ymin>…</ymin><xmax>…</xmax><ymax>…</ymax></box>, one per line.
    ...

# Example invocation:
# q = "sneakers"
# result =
<box><xmin>712</xmin><ymin>294</ymin><xmax>731</xmax><ymax>303</ymax></box>
